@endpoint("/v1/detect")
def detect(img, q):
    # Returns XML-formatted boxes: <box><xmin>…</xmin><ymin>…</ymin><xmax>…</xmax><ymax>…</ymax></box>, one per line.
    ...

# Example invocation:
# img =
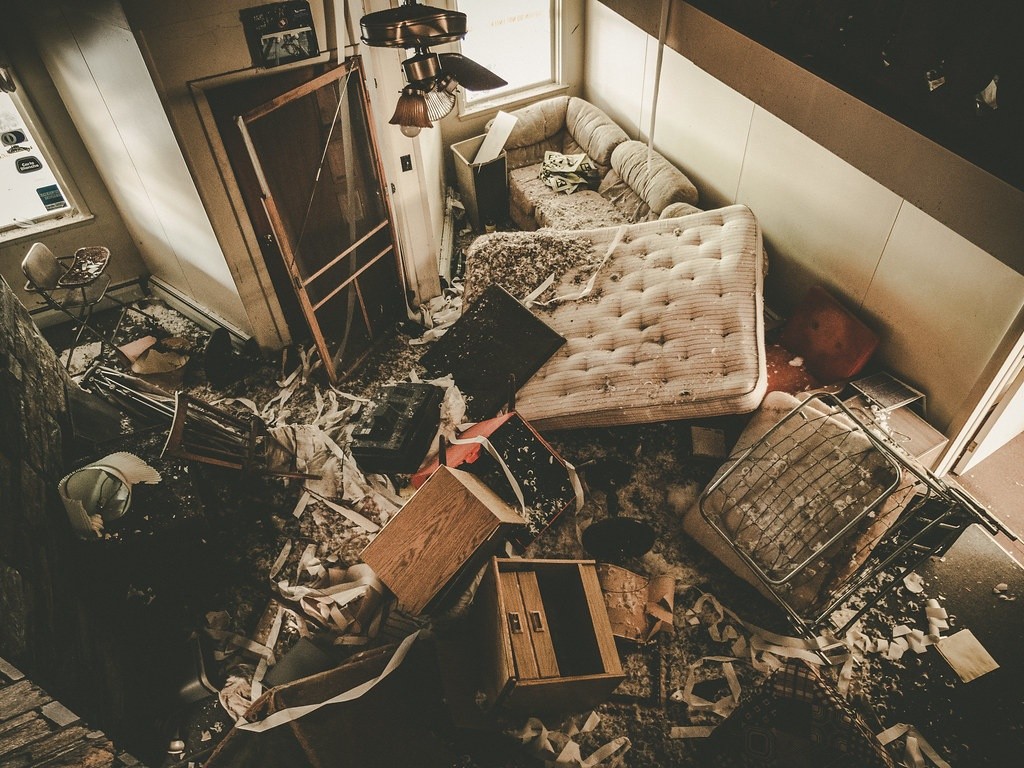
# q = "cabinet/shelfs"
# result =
<box><xmin>469</xmin><ymin>556</ymin><xmax>626</xmax><ymax>730</ymax></box>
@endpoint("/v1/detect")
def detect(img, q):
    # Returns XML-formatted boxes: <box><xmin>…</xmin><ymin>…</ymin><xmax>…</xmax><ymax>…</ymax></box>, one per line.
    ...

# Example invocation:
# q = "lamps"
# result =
<box><xmin>359</xmin><ymin>0</ymin><xmax>508</xmax><ymax>137</ymax></box>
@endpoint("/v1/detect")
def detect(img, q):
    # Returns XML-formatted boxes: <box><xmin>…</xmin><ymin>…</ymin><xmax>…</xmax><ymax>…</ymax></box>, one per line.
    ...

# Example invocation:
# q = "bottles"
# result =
<box><xmin>455</xmin><ymin>249</ymin><xmax>465</xmax><ymax>278</ymax></box>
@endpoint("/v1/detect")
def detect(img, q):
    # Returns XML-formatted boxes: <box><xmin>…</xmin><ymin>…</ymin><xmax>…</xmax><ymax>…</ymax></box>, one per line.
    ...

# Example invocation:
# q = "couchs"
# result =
<box><xmin>486</xmin><ymin>94</ymin><xmax>704</xmax><ymax>232</ymax></box>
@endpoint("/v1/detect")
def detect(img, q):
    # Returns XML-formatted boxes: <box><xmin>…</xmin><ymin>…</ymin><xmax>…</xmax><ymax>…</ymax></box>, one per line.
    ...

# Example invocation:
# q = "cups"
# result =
<box><xmin>485</xmin><ymin>219</ymin><xmax>497</xmax><ymax>233</ymax></box>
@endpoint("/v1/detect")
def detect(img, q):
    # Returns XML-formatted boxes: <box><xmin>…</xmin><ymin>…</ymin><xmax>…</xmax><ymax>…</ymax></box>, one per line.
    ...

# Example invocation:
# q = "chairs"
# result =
<box><xmin>20</xmin><ymin>241</ymin><xmax>160</xmax><ymax>370</ymax></box>
<box><xmin>762</xmin><ymin>282</ymin><xmax>881</xmax><ymax>400</ymax></box>
